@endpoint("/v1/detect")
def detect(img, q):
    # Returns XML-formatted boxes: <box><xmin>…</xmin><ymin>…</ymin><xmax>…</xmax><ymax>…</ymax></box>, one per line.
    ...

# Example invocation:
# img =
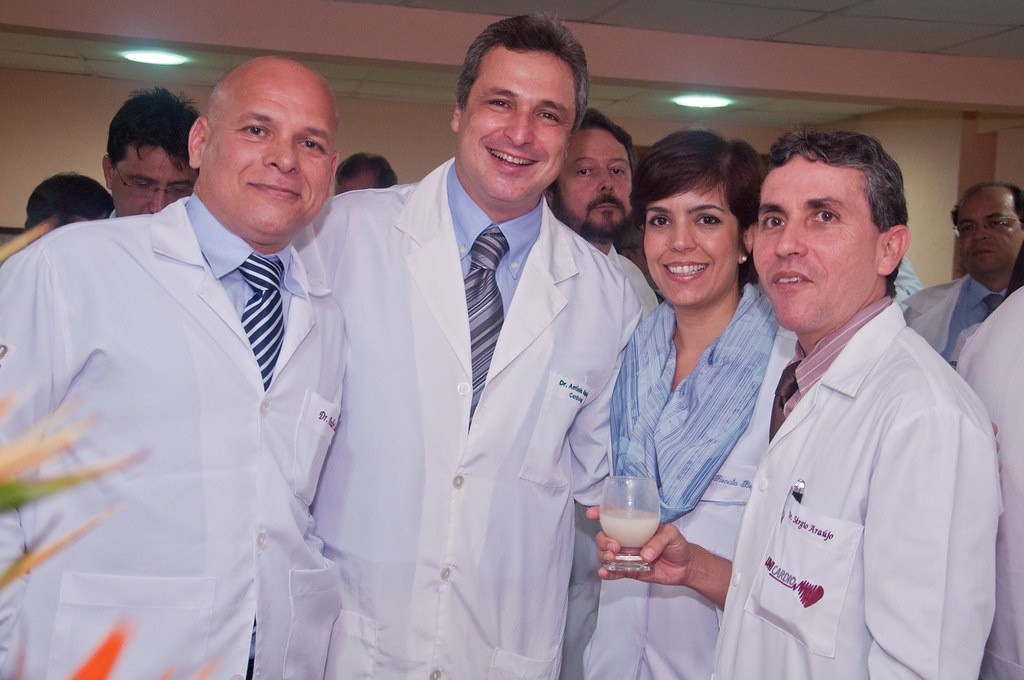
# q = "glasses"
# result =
<box><xmin>112</xmin><ymin>162</ymin><xmax>195</xmax><ymax>203</ymax></box>
<box><xmin>954</xmin><ymin>217</ymin><xmax>1022</xmax><ymax>239</ymax></box>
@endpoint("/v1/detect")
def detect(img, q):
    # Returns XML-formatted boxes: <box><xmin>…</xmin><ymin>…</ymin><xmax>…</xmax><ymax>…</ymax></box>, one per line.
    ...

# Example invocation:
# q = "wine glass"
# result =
<box><xmin>599</xmin><ymin>476</ymin><xmax>661</xmax><ymax>572</ymax></box>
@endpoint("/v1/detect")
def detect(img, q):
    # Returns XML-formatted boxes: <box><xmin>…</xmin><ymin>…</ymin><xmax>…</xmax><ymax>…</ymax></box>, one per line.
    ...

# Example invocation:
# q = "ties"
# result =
<box><xmin>769</xmin><ymin>360</ymin><xmax>802</xmax><ymax>445</ymax></box>
<box><xmin>238</xmin><ymin>254</ymin><xmax>285</xmax><ymax>391</ymax></box>
<box><xmin>983</xmin><ymin>294</ymin><xmax>1004</xmax><ymax>322</ymax></box>
<box><xmin>463</xmin><ymin>234</ymin><xmax>510</xmax><ymax>430</ymax></box>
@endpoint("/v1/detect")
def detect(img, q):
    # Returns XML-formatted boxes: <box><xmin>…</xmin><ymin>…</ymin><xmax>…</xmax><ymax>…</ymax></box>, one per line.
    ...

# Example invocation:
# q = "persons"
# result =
<box><xmin>0</xmin><ymin>54</ymin><xmax>350</xmax><ymax>679</ymax></box>
<box><xmin>586</xmin><ymin>129</ymin><xmax>1001</xmax><ymax>680</ymax></box>
<box><xmin>20</xmin><ymin>84</ymin><xmax>921</xmax><ymax>312</ymax></box>
<box><xmin>289</xmin><ymin>15</ymin><xmax>642</xmax><ymax>680</ymax></box>
<box><xmin>901</xmin><ymin>184</ymin><xmax>1024</xmax><ymax>680</ymax></box>
